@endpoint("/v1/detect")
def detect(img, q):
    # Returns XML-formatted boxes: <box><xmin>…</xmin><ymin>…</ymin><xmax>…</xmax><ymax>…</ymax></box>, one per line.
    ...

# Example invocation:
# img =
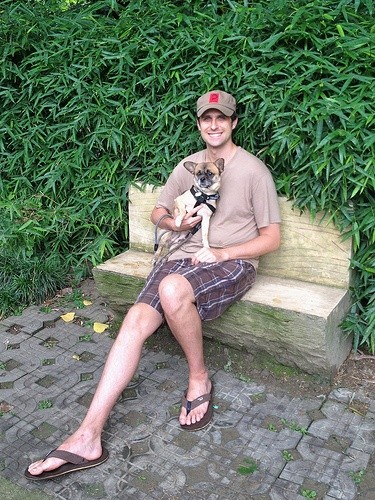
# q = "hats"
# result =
<box><xmin>197</xmin><ymin>90</ymin><xmax>236</xmax><ymax>118</ymax></box>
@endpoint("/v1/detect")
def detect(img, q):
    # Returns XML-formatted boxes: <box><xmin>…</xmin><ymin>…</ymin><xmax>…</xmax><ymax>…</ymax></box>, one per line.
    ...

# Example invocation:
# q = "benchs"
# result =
<box><xmin>91</xmin><ymin>182</ymin><xmax>358</xmax><ymax>377</ymax></box>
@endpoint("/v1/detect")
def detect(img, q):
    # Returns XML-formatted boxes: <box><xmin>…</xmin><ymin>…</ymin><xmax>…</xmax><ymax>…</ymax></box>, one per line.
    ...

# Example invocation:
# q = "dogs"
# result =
<box><xmin>151</xmin><ymin>158</ymin><xmax>226</xmax><ymax>268</ymax></box>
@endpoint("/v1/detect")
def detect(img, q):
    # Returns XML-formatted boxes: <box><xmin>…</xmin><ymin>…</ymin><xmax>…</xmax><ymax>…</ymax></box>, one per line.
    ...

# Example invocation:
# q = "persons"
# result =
<box><xmin>23</xmin><ymin>90</ymin><xmax>282</xmax><ymax>481</ymax></box>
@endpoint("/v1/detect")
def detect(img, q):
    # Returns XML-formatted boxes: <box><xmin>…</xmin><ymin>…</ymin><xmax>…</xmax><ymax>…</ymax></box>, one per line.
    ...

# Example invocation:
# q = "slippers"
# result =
<box><xmin>179</xmin><ymin>380</ymin><xmax>213</xmax><ymax>432</ymax></box>
<box><xmin>24</xmin><ymin>446</ymin><xmax>108</xmax><ymax>480</ymax></box>
